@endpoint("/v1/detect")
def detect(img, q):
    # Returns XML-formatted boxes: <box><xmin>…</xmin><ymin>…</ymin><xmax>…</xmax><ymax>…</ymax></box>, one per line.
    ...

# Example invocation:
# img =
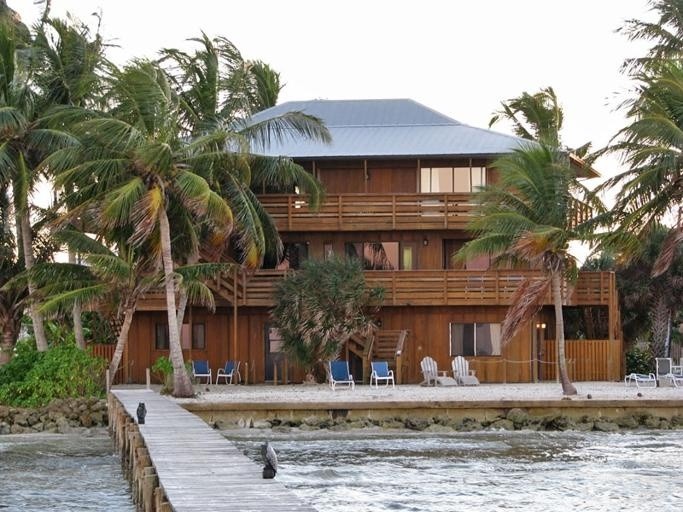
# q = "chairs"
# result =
<box><xmin>190</xmin><ymin>359</ymin><xmax>241</xmax><ymax>385</ymax></box>
<box><xmin>653</xmin><ymin>357</ymin><xmax>682</xmax><ymax>389</ymax></box>
<box><xmin>327</xmin><ymin>354</ymin><xmax>482</xmax><ymax>391</ymax></box>
